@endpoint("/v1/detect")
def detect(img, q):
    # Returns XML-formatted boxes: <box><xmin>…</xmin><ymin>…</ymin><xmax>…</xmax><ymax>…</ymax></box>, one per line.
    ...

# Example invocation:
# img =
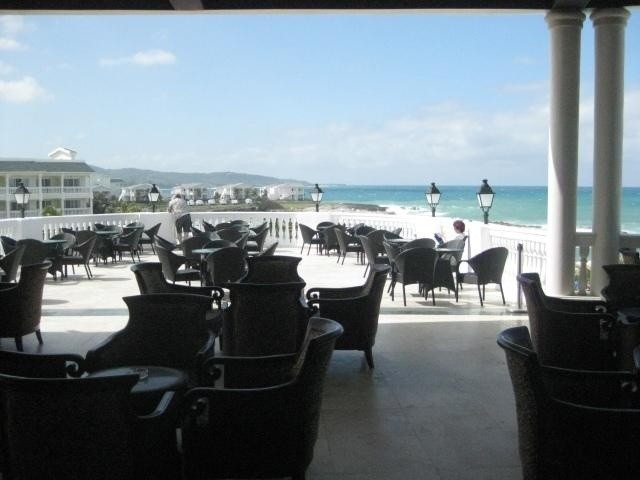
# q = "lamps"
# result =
<box><xmin>424</xmin><ymin>182</ymin><xmax>442</xmax><ymax>217</ymax></box>
<box><xmin>310</xmin><ymin>184</ymin><xmax>325</xmax><ymax>212</ymax></box>
<box><xmin>475</xmin><ymin>179</ymin><xmax>496</xmax><ymax>225</ymax></box>
<box><xmin>13</xmin><ymin>182</ymin><xmax>32</xmax><ymax>218</ymax></box>
<box><xmin>147</xmin><ymin>184</ymin><xmax>161</xmax><ymax>212</ymax></box>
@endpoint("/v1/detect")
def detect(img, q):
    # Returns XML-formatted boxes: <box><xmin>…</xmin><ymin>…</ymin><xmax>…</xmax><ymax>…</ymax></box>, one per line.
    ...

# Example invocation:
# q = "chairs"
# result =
<box><xmin>381</xmin><ymin>226</ymin><xmax>511</xmax><ymax>308</ymax></box>
<box><xmin>0</xmin><ymin>221</ymin><xmax>392</xmax><ymax>480</ymax></box>
<box><xmin>494</xmin><ymin>245</ymin><xmax>640</xmax><ymax>480</ymax></box>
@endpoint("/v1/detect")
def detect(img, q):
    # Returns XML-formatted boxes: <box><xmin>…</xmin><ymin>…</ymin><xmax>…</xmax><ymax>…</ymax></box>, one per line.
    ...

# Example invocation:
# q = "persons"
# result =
<box><xmin>167</xmin><ymin>192</ymin><xmax>191</xmax><ymax>244</ymax></box>
<box><xmin>436</xmin><ymin>219</ymin><xmax>465</xmax><ymax>264</ymax></box>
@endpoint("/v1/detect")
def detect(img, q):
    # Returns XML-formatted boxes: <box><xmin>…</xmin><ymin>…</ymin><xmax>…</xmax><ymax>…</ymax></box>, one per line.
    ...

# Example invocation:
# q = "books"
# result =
<box><xmin>435</xmin><ymin>234</ymin><xmax>446</xmax><ymax>246</ymax></box>
